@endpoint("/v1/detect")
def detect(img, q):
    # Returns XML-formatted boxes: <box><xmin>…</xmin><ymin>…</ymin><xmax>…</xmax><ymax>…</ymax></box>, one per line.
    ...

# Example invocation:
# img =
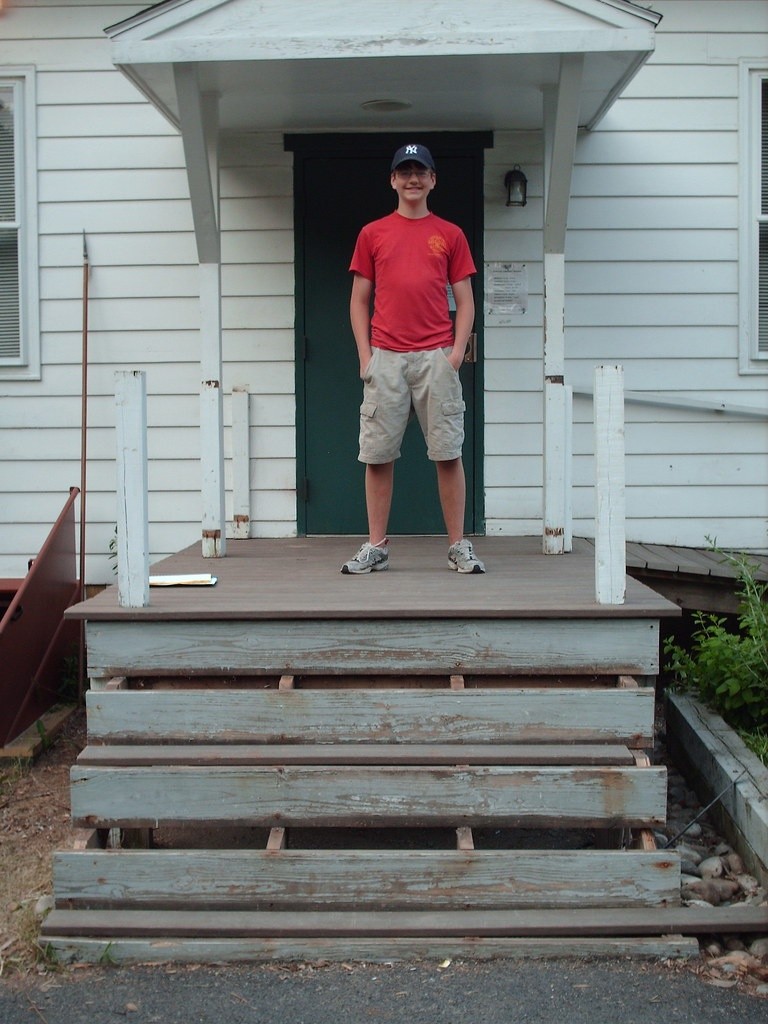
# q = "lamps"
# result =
<box><xmin>504</xmin><ymin>163</ymin><xmax>528</xmax><ymax>207</ymax></box>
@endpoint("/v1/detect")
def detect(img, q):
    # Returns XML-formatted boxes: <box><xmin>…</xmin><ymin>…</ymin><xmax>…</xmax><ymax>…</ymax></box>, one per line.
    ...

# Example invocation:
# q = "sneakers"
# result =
<box><xmin>448</xmin><ymin>538</ymin><xmax>486</xmax><ymax>573</ymax></box>
<box><xmin>341</xmin><ymin>538</ymin><xmax>390</xmax><ymax>574</ymax></box>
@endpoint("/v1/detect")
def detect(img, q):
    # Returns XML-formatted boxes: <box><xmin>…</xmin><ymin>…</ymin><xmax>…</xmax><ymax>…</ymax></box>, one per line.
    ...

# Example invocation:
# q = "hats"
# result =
<box><xmin>391</xmin><ymin>144</ymin><xmax>435</xmax><ymax>172</ymax></box>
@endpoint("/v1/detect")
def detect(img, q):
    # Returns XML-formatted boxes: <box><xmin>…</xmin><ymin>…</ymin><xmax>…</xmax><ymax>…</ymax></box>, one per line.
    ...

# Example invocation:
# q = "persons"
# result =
<box><xmin>341</xmin><ymin>143</ymin><xmax>486</xmax><ymax>575</ymax></box>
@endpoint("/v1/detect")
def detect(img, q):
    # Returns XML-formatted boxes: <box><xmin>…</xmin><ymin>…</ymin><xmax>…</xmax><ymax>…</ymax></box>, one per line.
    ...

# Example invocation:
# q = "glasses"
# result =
<box><xmin>394</xmin><ymin>169</ymin><xmax>432</xmax><ymax>180</ymax></box>
